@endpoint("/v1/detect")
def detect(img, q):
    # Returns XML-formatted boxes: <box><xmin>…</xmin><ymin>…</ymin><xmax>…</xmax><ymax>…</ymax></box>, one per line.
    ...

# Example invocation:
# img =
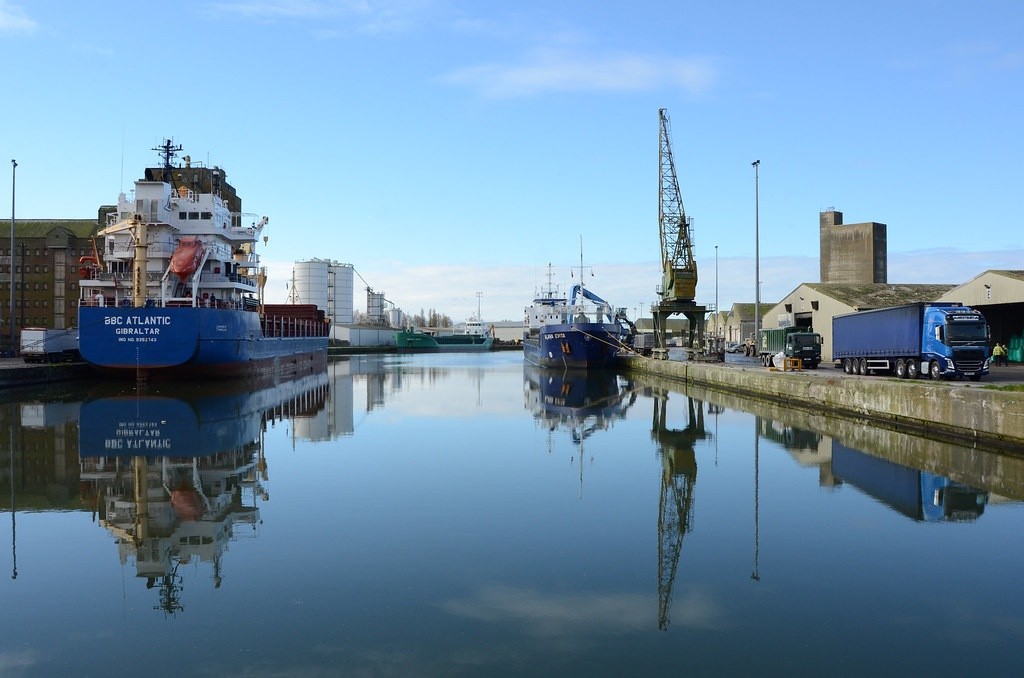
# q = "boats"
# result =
<box><xmin>78</xmin><ymin>366</ymin><xmax>331</xmax><ymax>619</ymax></box>
<box><xmin>463</xmin><ymin>292</ymin><xmax>492</xmax><ymax>338</ymax></box>
<box><xmin>523</xmin><ymin>235</ymin><xmax>621</xmax><ymax>369</ymax></box>
<box><xmin>523</xmin><ymin>360</ymin><xmax>637</xmax><ymax>499</ymax></box>
<box><xmin>78</xmin><ymin>129</ymin><xmax>332</xmax><ymax>379</ymax></box>
<box><xmin>393</xmin><ymin>325</ymin><xmax>494</xmax><ymax>353</ymax></box>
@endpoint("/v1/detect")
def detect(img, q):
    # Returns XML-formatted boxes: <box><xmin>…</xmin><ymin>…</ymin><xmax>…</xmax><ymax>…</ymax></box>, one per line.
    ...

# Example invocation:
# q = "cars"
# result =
<box><xmin>728</xmin><ymin>344</ymin><xmax>743</xmax><ymax>353</ymax></box>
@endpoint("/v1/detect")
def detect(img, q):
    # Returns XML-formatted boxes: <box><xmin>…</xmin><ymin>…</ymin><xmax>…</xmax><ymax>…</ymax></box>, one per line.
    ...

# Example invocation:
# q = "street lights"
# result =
<box><xmin>752</xmin><ymin>160</ymin><xmax>760</xmax><ymax>341</ymax></box>
<box><xmin>750</xmin><ymin>417</ymin><xmax>761</xmax><ymax>581</ymax></box>
<box><xmin>639</xmin><ymin>303</ymin><xmax>643</xmax><ymax>334</ymax></box>
<box><xmin>715</xmin><ymin>246</ymin><xmax>717</xmax><ymax>334</ymax></box>
<box><xmin>10</xmin><ymin>158</ymin><xmax>18</xmax><ymax>350</ymax></box>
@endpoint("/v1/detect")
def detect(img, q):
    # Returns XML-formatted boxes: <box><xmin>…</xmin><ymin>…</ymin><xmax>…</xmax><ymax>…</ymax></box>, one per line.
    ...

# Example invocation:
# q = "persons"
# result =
<box><xmin>993</xmin><ymin>343</ymin><xmax>1006</xmax><ymax>367</ymax></box>
<box><xmin>210</xmin><ymin>293</ymin><xmax>215</xmax><ymax>306</ymax></box>
<box><xmin>1002</xmin><ymin>344</ymin><xmax>1009</xmax><ymax>367</ymax></box>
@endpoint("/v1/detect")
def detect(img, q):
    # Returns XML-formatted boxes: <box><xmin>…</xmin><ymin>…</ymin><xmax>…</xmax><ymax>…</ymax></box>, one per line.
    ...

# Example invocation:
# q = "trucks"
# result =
<box><xmin>633</xmin><ymin>334</ymin><xmax>654</xmax><ymax>356</ymax></box>
<box><xmin>759</xmin><ymin>326</ymin><xmax>823</xmax><ymax>369</ymax></box>
<box><xmin>20</xmin><ymin>327</ymin><xmax>78</xmax><ymax>363</ymax></box>
<box><xmin>743</xmin><ymin>333</ymin><xmax>755</xmax><ymax>357</ymax></box>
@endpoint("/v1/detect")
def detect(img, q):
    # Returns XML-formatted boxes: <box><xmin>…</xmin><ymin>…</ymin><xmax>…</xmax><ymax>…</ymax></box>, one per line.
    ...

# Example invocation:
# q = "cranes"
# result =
<box><xmin>650</xmin><ymin>391</ymin><xmax>714</xmax><ymax>633</ymax></box>
<box><xmin>234</xmin><ymin>216</ymin><xmax>269</xmax><ymax>276</ymax></box>
<box><xmin>651</xmin><ymin>104</ymin><xmax>713</xmax><ymax>360</ymax></box>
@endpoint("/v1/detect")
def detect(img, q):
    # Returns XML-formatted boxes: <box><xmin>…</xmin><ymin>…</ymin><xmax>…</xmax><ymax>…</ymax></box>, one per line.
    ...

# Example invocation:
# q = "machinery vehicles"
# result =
<box><xmin>615</xmin><ymin>307</ymin><xmax>640</xmax><ymax>349</ymax></box>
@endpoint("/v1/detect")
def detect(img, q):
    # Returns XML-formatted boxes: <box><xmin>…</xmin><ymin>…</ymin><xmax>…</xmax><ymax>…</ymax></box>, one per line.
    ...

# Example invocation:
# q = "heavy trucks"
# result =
<box><xmin>831</xmin><ymin>439</ymin><xmax>989</xmax><ymax>523</ymax></box>
<box><xmin>832</xmin><ymin>302</ymin><xmax>991</xmax><ymax>381</ymax></box>
